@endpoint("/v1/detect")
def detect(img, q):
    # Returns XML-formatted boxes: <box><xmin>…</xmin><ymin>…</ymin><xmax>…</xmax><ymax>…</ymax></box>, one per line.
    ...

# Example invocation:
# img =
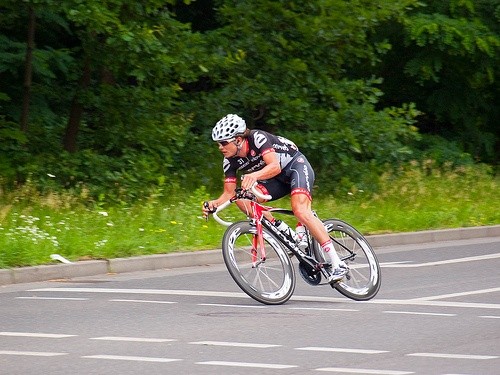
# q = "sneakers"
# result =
<box><xmin>286</xmin><ymin>239</ymin><xmax>308</xmax><ymax>257</ymax></box>
<box><xmin>328</xmin><ymin>261</ymin><xmax>349</xmax><ymax>284</ymax></box>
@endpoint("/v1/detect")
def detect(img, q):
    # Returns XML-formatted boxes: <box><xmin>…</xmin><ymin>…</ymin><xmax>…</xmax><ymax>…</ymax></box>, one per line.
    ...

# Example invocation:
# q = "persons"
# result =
<box><xmin>201</xmin><ymin>113</ymin><xmax>348</xmax><ymax>283</ymax></box>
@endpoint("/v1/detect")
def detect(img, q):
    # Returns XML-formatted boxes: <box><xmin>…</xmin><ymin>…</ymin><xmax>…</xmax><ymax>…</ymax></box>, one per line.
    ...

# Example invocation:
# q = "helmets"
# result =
<box><xmin>212</xmin><ymin>114</ymin><xmax>246</xmax><ymax>141</ymax></box>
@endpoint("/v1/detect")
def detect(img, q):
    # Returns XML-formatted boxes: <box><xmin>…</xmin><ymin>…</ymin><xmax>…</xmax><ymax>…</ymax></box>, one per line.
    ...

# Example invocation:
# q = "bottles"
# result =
<box><xmin>296</xmin><ymin>221</ymin><xmax>308</xmax><ymax>248</ymax></box>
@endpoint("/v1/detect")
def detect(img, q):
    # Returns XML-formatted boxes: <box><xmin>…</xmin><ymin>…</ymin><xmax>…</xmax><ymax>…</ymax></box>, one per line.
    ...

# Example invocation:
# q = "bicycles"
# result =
<box><xmin>204</xmin><ymin>176</ymin><xmax>382</xmax><ymax>306</ymax></box>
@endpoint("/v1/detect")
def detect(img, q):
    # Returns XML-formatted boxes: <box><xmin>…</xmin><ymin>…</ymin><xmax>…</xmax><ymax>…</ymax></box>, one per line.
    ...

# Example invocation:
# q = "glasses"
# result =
<box><xmin>216</xmin><ymin>138</ymin><xmax>235</xmax><ymax>146</ymax></box>
